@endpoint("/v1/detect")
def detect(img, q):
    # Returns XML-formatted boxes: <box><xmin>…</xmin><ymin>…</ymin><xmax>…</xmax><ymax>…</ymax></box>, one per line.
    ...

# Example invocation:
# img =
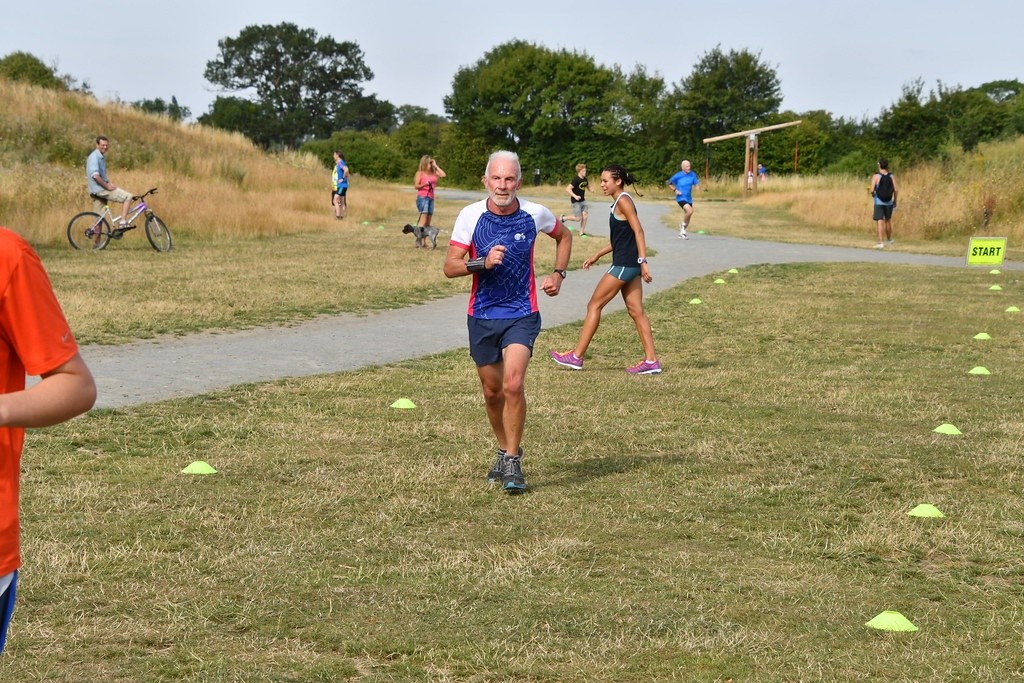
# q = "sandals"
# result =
<box><xmin>93</xmin><ymin>245</ymin><xmax>106</xmax><ymax>250</ymax></box>
<box><xmin>118</xmin><ymin>222</ymin><xmax>136</xmax><ymax>229</ymax></box>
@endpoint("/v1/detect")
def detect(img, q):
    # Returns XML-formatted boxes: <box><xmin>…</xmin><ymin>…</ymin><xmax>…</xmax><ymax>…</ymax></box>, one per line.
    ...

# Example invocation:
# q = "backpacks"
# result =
<box><xmin>875</xmin><ymin>172</ymin><xmax>894</xmax><ymax>203</ymax></box>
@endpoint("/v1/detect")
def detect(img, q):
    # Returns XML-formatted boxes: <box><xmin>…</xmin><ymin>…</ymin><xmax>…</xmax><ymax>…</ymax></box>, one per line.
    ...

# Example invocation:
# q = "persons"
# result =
<box><xmin>757</xmin><ymin>164</ymin><xmax>766</xmax><ymax>191</ymax></box>
<box><xmin>870</xmin><ymin>158</ymin><xmax>898</xmax><ymax>248</ymax></box>
<box><xmin>0</xmin><ymin>226</ymin><xmax>97</xmax><ymax>660</ymax></box>
<box><xmin>666</xmin><ymin>160</ymin><xmax>700</xmax><ymax>240</ymax></box>
<box><xmin>87</xmin><ymin>136</ymin><xmax>136</xmax><ymax>251</ymax></box>
<box><xmin>551</xmin><ymin>165</ymin><xmax>663</xmax><ymax>376</ymax></box>
<box><xmin>560</xmin><ymin>164</ymin><xmax>596</xmax><ymax>239</ymax></box>
<box><xmin>444</xmin><ymin>150</ymin><xmax>572</xmax><ymax>494</ymax></box>
<box><xmin>331</xmin><ymin>150</ymin><xmax>349</xmax><ymax>220</ymax></box>
<box><xmin>414</xmin><ymin>155</ymin><xmax>446</xmax><ymax>247</ymax></box>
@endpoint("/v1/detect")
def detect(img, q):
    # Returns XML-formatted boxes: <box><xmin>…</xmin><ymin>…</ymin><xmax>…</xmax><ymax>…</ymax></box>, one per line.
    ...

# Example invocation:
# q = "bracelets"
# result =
<box><xmin>673</xmin><ymin>188</ymin><xmax>677</xmax><ymax>192</ymax></box>
<box><xmin>467</xmin><ymin>257</ymin><xmax>485</xmax><ymax>271</ymax></box>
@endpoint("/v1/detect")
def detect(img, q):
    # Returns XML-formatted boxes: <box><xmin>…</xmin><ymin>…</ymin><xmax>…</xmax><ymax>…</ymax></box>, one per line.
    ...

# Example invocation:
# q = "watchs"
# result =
<box><xmin>638</xmin><ymin>258</ymin><xmax>648</xmax><ymax>264</ymax></box>
<box><xmin>554</xmin><ymin>269</ymin><xmax>566</xmax><ymax>279</ymax></box>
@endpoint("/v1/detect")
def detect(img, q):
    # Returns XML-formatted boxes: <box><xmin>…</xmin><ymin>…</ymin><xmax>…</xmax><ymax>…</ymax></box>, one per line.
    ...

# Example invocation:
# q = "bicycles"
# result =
<box><xmin>68</xmin><ymin>186</ymin><xmax>173</xmax><ymax>254</ymax></box>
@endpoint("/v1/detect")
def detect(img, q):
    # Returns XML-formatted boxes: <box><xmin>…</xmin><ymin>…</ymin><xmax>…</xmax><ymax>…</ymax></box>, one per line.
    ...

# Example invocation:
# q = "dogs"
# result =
<box><xmin>402</xmin><ymin>223</ymin><xmax>448</xmax><ymax>250</ymax></box>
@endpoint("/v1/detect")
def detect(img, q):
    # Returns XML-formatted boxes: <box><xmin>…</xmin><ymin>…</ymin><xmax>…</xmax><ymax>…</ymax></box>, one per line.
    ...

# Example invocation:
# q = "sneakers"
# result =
<box><xmin>551</xmin><ymin>349</ymin><xmax>583</xmax><ymax>370</ymax></box>
<box><xmin>503</xmin><ymin>453</ymin><xmax>525</xmax><ymax>490</ymax></box>
<box><xmin>627</xmin><ymin>361</ymin><xmax>661</xmax><ymax>375</ymax></box>
<box><xmin>486</xmin><ymin>447</ymin><xmax>522</xmax><ymax>483</ymax></box>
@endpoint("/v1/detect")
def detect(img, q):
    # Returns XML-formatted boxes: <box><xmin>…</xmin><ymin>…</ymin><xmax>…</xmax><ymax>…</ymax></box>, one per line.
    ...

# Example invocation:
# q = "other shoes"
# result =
<box><xmin>889</xmin><ymin>239</ymin><xmax>894</xmax><ymax>243</ymax></box>
<box><xmin>579</xmin><ymin>233</ymin><xmax>587</xmax><ymax>238</ymax></box>
<box><xmin>678</xmin><ymin>233</ymin><xmax>688</xmax><ymax>240</ymax></box>
<box><xmin>560</xmin><ymin>214</ymin><xmax>565</xmax><ymax>224</ymax></box>
<box><xmin>874</xmin><ymin>242</ymin><xmax>884</xmax><ymax>248</ymax></box>
<box><xmin>681</xmin><ymin>224</ymin><xmax>687</xmax><ymax>234</ymax></box>
